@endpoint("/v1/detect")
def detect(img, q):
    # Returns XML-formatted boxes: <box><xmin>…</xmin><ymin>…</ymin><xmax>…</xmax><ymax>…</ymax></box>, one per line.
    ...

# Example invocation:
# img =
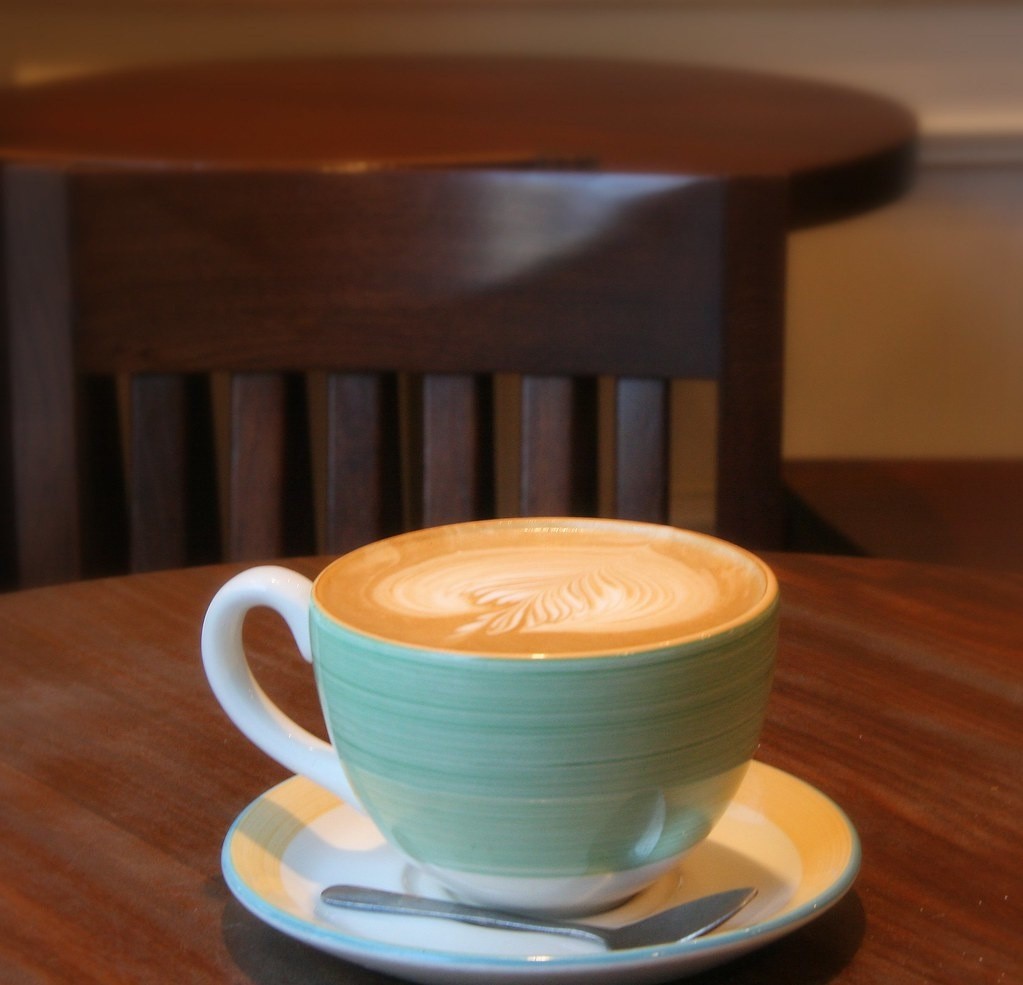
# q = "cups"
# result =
<box><xmin>201</xmin><ymin>516</ymin><xmax>780</xmax><ymax>923</ymax></box>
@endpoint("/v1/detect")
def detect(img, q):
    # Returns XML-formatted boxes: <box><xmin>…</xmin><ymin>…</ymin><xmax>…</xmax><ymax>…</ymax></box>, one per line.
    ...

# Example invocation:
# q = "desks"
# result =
<box><xmin>0</xmin><ymin>548</ymin><xmax>1023</xmax><ymax>983</ymax></box>
<box><xmin>3</xmin><ymin>50</ymin><xmax>918</xmax><ymax>545</ymax></box>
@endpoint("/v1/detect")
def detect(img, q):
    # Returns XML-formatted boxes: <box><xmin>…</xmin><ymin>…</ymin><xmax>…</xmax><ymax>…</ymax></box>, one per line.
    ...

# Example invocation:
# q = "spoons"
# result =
<box><xmin>319</xmin><ymin>887</ymin><xmax>759</xmax><ymax>951</ymax></box>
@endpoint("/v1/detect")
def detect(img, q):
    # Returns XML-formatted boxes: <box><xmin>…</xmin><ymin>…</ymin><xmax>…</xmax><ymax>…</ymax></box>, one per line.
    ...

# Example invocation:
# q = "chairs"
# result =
<box><xmin>0</xmin><ymin>170</ymin><xmax>718</xmax><ymax>593</ymax></box>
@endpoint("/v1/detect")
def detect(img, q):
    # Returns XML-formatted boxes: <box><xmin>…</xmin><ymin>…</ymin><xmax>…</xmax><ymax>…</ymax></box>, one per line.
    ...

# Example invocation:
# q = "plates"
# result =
<box><xmin>220</xmin><ymin>757</ymin><xmax>861</xmax><ymax>985</ymax></box>
